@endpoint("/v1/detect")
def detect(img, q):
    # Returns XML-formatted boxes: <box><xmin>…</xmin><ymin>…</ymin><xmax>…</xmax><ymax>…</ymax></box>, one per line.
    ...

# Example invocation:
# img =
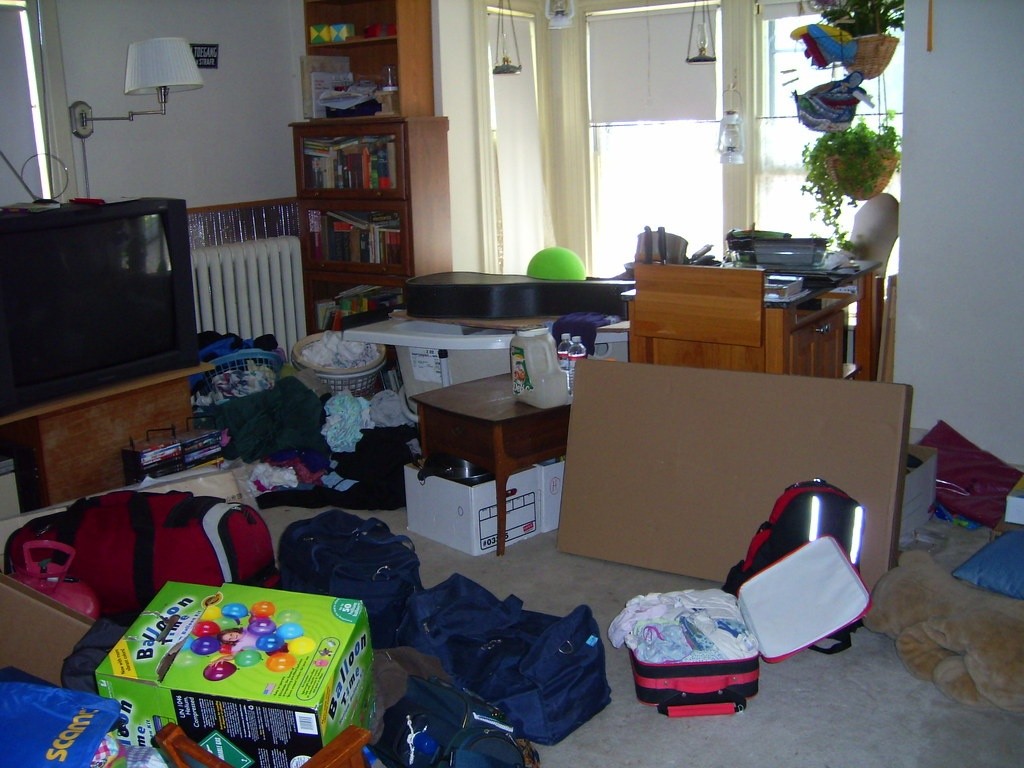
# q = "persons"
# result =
<box><xmin>208</xmin><ymin>626</ymin><xmax>269</xmax><ymax>668</ymax></box>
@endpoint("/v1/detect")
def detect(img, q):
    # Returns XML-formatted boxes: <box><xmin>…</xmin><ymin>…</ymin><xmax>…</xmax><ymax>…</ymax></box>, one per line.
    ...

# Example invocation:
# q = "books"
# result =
<box><xmin>379</xmin><ymin>360</ymin><xmax>404</xmax><ymax>393</ymax></box>
<box><xmin>308</xmin><ymin>210</ymin><xmax>401</xmax><ymax>264</ymax></box>
<box><xmin>300</xmin><ymin>55</ymin><xmax>354</xmax><ymax>119</ymax></box>
<box><xmin>316</xmin><ymin>287</ymin><xmax>403</xmax><ymax>330</ymax></box>
<box><xmin>303</xmin><ymin>135</ymin><xmax>396</xmax><ymax>189</ymax></box>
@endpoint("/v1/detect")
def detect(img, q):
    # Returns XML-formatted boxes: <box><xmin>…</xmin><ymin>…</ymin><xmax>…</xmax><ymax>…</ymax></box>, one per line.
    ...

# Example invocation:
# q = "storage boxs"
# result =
<box><xmin>331</xmin><ymin>22</ymin><xmax>354</xmax><ymax>41</ymax></box>
<box><xmin>402</xmin><ymin>355</ymin><xmax>1023</xmax><ymax>598</ymax></box>
<box><xmin>0</xmin><ymin>569</ymin><xmax>385</xmax><ymax>768</ymax></box>
<box><xmin>309</xmin><ymin>22</ymin><xmax>329</xmax><ymax>44</ymax></box>
<box><xmin>304</xmin><ymin>0</ymin><xmax>431</xmax><ymax>116</ymax></box>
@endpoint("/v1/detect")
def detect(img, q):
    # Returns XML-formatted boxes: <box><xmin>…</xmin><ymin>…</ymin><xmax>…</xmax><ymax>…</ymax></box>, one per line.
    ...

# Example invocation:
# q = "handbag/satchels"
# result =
<box><xmin>379</xmin><ymin>675</ymin><xmax>527</xmax><ymax>768</ymax></box>
<box><xmin>277</xmin><ymin>510</ymin><xmax>425</xmax><ymax>649</ymax></box>
<box><xmin>5</xmin><ymin>491</ymin><xmax>280</xmax><ymax>617</ymax></box>
<box><xmin>396</xmin><ymin>573</ymin><xmax>612</xmax><ymax>744</ymax></box>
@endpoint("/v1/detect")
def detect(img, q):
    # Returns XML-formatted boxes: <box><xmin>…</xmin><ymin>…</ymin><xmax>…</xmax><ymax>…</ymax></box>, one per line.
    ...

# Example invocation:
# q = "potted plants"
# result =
<box><xmin>802</xmin><ymin>118</ymin><xmax>901</xmax><ymax>257</ymax></box>
<box><xmin>812</xmin><ymin>0</ymin><xmax>905</xmax><ymax>81</ymax></box>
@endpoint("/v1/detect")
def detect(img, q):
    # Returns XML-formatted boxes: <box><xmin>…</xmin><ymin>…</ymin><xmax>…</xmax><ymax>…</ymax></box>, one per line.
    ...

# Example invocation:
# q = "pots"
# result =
<box><xmin>623</xmin><ymin>224</ymin><xmax>722</xmax><ymax>276</ymax></box>
<box><xmin>412</xmin><ymin>454</ymin><xmax>491</xmax><ymax>487</ymax></box>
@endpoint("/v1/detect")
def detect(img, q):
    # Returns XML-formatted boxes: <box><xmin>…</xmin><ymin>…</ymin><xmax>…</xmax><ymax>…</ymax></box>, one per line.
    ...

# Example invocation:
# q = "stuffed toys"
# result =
<box><xmin>862</xmin><ymin>550</ymin><xmax>1024</xmax><ymax>714</ymax></box>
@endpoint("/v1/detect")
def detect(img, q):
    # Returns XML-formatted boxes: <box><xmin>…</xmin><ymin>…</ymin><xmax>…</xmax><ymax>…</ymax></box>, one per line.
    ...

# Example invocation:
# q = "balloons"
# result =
<box><xmin>190</xmin><ymin>601</ymin><xmax>316</xmax><ymax>682</ymax></box>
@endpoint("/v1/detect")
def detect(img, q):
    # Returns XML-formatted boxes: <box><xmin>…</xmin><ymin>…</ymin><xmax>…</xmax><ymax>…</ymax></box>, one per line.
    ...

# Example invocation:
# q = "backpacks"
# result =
<box><xmin>724</xmin><ymin>481</ymin><xmax>865</xmax><ymax>656</ymax></box>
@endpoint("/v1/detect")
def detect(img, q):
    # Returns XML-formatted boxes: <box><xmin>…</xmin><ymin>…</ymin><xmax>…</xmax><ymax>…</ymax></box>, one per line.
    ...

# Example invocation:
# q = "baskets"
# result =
<box><xmin>842</xmin><ymin>35</ymin><xmax>899</xmax><ymax>79</ymax></box>
<box><xmin>193</xmin><ymin>351</ymin><xmax>282</xmax><ymax>407</ymax></box>
<box><xmin>827</xmin><ymin>148</ymin><xmax>898</xmax><ymax>201</ymax></box>
<box><xmin>291</xmin><ymin>332</ymin><xmax>386</xmax><ymax>397</ymax></box>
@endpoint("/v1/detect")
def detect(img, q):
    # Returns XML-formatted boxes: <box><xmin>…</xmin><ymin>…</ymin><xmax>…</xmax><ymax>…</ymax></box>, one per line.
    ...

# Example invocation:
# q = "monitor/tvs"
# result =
<box><xmin>0</xmin><ymin>196</ymin><xmax>200</xmax><ymax>415</ymax></box>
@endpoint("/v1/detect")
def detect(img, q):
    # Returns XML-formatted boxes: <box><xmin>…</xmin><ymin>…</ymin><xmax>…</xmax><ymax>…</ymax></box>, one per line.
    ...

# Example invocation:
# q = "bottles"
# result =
<box><xmin>381</xmin><ymin>64</ymin><xmax>398</xmax><ymax>91</ymax></box>
<box><xmin>557</xmin><ymin>333</ymin><xmax>586</xmax><ymax>395</ymax></box>
<box><xmin>510</xmin><ymin>327</ymin><xmax>569</xmax><ymax>408</ymax></box>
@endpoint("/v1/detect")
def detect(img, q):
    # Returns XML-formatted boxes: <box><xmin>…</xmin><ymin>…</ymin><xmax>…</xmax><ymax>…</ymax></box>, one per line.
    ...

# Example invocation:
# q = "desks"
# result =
<box><xmin>407</xmin><ymin>372</ymin><xmax>574</xmax><ymax>557</ymax></box>
<box><xmin>0</xmin><ymin>363</ymin><xmax>213</xmax><ymax>508</ymax></box>
<box><xmin>343</xmin><ymin>319</ymin><xmax>627</xmax><ymax>389</ymax></box>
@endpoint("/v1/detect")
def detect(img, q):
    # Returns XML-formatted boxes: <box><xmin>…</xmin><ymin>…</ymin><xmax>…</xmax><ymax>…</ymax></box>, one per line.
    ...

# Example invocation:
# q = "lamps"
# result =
<box><xmin>70</xmin><ymin>36</ymin><xmax>202</xmax><ymax>138</ymax></box>
<box><xmin>686</xmin><ymin>1</ymin><xmax>716</xmax><ymax>65</ymax></box>
<box><xmin>492</xmin><ymin>0</ymin><xmax>522</xmax><ymax>75</ymax></box>
<box><xmin>717</xmin><ymin>0</ymin><xmax>745</xmax><ymax>166</ymax></box>
<box><xmin>544</xmin><ymin>0</ymin><xmax>574</xmax><ymax>29</ymax></box>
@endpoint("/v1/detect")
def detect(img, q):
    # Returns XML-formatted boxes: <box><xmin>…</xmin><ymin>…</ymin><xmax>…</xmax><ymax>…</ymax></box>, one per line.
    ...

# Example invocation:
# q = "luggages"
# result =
<box><xmin>629</xmin><ymin>534</ymin><xmax>874</xmax><ymax>718</ymax></box>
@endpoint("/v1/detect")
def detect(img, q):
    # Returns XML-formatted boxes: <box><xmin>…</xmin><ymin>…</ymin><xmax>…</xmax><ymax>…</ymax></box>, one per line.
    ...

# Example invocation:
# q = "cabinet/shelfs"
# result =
<box><xmin>288</xmin><ymin>116</ymin><xmax>449</xmax><ymax>333</ymax></box>
<box><xmin>617</xmin><ymin>259</ymin><xmax>886</xmax><ymax>375</ymax></box>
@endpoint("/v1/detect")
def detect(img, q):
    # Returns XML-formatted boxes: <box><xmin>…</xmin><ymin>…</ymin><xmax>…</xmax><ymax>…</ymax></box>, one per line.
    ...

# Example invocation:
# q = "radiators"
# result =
<box><xmin>190</xmin><ymin>235</ymin><xmax>308</xmax><ymax>363</ymax></box>
